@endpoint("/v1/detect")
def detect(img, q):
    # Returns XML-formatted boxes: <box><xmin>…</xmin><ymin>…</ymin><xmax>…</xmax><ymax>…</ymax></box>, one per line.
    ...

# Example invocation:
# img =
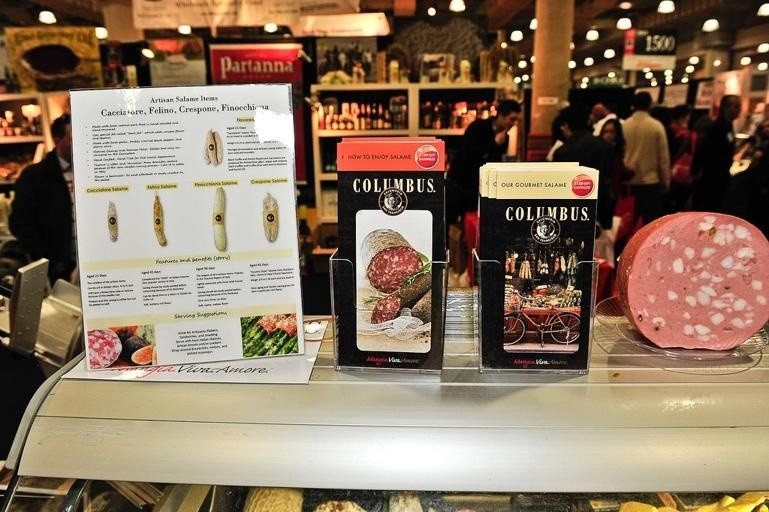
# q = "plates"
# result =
<box><xmin>594</xmin><ymin>293</ymin><xmax>769</xmax><ymax>363</ymax></box>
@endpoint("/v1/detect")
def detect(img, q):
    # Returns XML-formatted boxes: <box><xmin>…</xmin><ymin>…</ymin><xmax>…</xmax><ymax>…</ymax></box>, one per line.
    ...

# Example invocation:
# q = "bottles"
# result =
<box><xmin>317</xmin><ymin>102</ymin><xmax>408</xmax><ymax>130</ymax></box>
<box><xmin>0</xmin><ymin>60</ymin><xmax>43</xmax><ymax>136</ymax></box>
<box><xmin>421</xmin><ymin>100</ymin><xmax>497</xmax><ymax>128</ymax></box>
<box><xmin>335</xmin><ymin>51</ymin><xmax>474</xmax><ymax>83</ymax></box>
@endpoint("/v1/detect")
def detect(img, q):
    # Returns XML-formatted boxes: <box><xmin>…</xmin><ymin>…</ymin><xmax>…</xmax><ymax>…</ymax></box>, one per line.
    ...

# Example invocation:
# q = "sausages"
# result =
<box><xmin>505</xmin><ymin>253</ymin><xmax>578</xmax><ymax>279</ymax></box>
<box><xmin>615</xmin><ymin>212</ymin><xmax>769</xmax><ymax>354</ymax></box>
<box><xmin>361</xmin><ymin>228</ymin><xmax>431</xmax><ymax>324</ymax></box>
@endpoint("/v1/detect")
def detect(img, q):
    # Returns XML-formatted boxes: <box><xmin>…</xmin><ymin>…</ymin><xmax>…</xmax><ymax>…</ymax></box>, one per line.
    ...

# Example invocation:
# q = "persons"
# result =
<box><xmin>546</xmin><ymin>90</ymin><xmax>769</xmax><ymax>232</ymax></box>
<box><xmin>8</xmin><ymin>112</ymin><xmax>75</xmax><ymax>289</ymax></box>
<box><xmin>446</xmin><ymin>99</ymin><xmax>521</xmax><ymax>249</ymax></box>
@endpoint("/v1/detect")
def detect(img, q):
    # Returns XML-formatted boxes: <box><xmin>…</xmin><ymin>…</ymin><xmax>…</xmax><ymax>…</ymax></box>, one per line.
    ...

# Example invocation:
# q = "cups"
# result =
<box><xmin>105</xmin><ymin>62</ymin><xmax>138</xmax><ymax>87</ymax></box>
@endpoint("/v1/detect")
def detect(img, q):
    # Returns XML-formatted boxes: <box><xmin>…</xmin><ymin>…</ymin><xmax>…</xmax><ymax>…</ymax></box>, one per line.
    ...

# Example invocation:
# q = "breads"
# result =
<box><xmin>77</xmin><ymin>486</ymin><xmax>768</xmax><ymax>512</ymax></box>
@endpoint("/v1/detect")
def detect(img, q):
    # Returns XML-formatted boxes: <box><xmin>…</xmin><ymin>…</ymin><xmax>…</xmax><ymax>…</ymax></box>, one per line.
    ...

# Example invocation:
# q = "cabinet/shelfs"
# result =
<box><xmin>297</xmin><ymin>73</ymin><xmax>529</xmax><ymax>252</ymax></box>
<box><xmin>0</xmin><ymin>311</ymin><xmax>769</xmax><ymax>512</ymax></box>
<box><xmin>3</xmin><ymin>86</ymin><xmax>56</xmax><ymax>260</ymax></box>
